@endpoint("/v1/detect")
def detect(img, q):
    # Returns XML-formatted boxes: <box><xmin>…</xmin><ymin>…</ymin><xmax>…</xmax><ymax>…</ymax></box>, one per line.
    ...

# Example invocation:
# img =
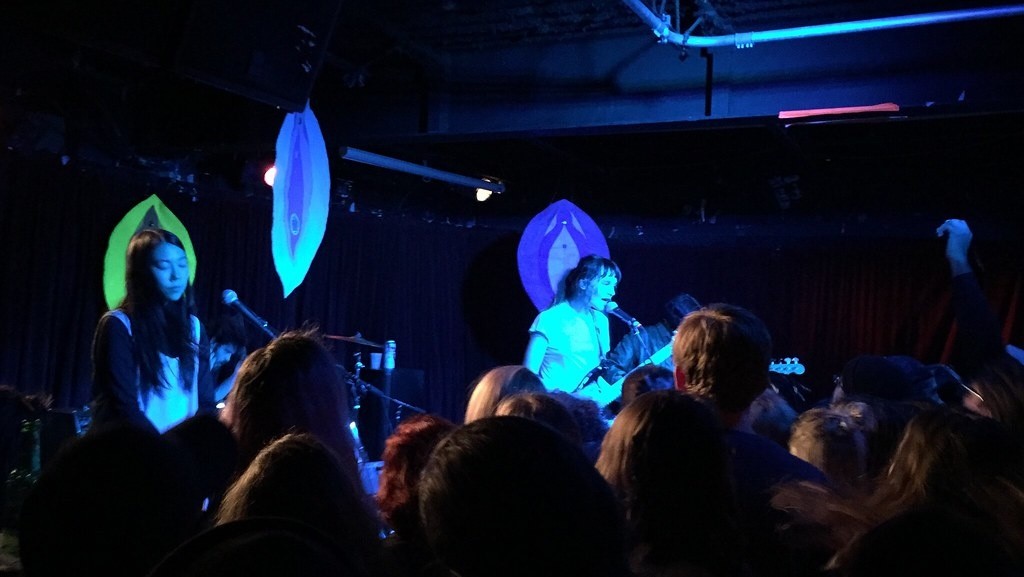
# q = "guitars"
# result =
<box><xmin>571</xmin><ymin>331</ymin><xmax>679</xmax><ymax>428</ymax></box>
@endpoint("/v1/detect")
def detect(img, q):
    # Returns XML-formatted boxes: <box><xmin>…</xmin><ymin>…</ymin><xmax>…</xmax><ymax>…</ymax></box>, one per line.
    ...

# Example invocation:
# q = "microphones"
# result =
<box><xmin>605</xmin><ymin>302</ymin><xmax>643</xmax><ymax>331</ymax></box>
<box><xmin>221</xmin><ymin>289</ymin><xmax>280</xmax><ymax>343</ymax></box>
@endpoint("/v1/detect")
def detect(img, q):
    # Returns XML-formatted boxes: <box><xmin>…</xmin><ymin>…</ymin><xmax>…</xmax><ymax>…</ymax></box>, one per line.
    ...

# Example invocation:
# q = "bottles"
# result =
<box><xmin>384</xmin><ymin>339</ymin><xmax>396</xmax><ymax>369</ymax></box>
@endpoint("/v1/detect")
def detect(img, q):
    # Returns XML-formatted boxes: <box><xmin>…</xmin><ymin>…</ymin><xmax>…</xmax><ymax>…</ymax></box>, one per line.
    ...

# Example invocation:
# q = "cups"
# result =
<box><xmin>369</xmin><ymin>351</ymin><xmax>382</xmax><ymax>370</ymax></box>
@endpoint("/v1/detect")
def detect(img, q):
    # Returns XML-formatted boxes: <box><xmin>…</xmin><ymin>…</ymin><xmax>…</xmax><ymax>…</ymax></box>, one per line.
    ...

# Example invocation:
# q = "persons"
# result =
<box><xmin>87</xmin><ymin>224</ymin><xmax>222</xmax><ymax>501</ymax></box>
<box><xmin>0</xmin><ymin>307</ymin><xmax>1023</xmax><ymax>576</ymax></box>
<box><xmin>577</xmin><ymin>291</ymin><xmax>708</xmax><ymax>414</ymax></box>
<box><xmin>937</xmin><ymin>209</ymin><xmax>1024</xmax><ymax>465</ymax></box>
<box><xmin>524</xmin><ymin>252</ymin><xmax>620</xmax><ymax>427</ymax></box>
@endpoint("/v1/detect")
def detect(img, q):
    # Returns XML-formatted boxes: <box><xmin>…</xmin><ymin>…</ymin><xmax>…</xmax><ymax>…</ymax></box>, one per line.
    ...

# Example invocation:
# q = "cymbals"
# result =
<box><xmin>324</xmin><ymin>335</ymin><xmax>384</xmax><ymax>348</ymax></box>
